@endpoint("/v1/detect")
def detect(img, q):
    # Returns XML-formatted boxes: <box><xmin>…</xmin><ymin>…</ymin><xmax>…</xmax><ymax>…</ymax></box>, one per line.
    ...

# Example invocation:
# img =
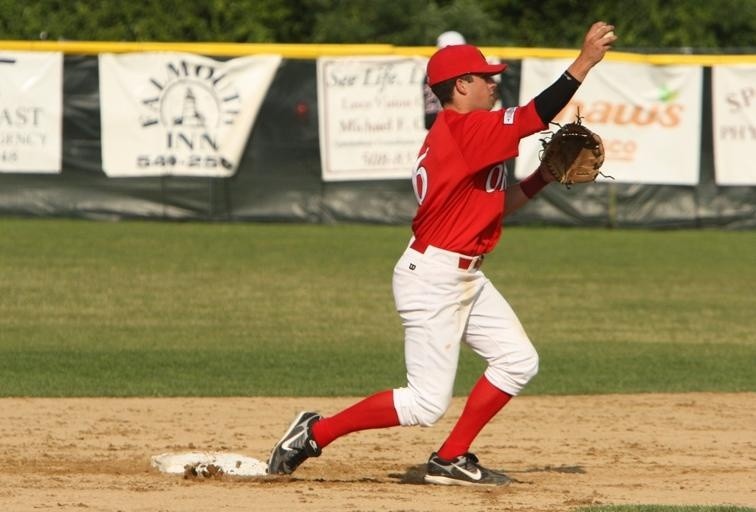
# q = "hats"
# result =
<box><xmin>427</xmin><ymin>44</ymin><xmax>509</xmax><ymax>87</ymax></box>
<box><xmin>437</xmin><ymin>30</ymin><xmax>466</xmax><ymax>50</ymax></box>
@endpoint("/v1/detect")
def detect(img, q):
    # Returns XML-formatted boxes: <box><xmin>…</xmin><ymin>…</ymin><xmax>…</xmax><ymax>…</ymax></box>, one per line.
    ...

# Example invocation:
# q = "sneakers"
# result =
<box><xmin>266</xmin><ymin>411</ymin><xmax>323</xmax><ymax>474</ymax></box>
<box><xmin>424</xmin><ymin>452</ymin><xmax>511</xmax><ymax>487</ymax></box>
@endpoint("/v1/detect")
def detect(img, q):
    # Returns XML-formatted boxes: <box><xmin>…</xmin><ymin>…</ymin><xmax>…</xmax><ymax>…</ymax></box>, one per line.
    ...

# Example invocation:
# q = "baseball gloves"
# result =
<box><xmin>543</xmin><ymin>124</ymin><xmax>605</xmax><ymax>184</ymax></box>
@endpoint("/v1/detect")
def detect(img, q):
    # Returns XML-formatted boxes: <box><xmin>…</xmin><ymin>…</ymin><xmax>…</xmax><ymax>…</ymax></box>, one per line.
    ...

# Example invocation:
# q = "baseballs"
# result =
<box><xmin>597</xmin><ymin>26</ymin><xmax>614</xmax><ymax>39</ymax></box>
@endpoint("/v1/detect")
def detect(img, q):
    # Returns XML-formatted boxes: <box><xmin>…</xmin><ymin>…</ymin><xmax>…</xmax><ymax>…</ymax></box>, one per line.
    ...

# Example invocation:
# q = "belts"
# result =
<box><xmin>410</xmin><ymin>240</ymin><xmax>484</xmax><ymax>269</ymax></box>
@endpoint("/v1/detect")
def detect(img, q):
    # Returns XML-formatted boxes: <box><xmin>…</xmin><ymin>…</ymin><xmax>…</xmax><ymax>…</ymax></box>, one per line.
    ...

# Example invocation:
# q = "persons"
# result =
<box><xmin>422</xmin><ymin>30</ymin><xmax>467</xmax><ymax>130</ymax></box>
<box><xmin>267</xmin><ymin>22</ymin><xmax>619</xmax><ymax>489</ymax></box>
